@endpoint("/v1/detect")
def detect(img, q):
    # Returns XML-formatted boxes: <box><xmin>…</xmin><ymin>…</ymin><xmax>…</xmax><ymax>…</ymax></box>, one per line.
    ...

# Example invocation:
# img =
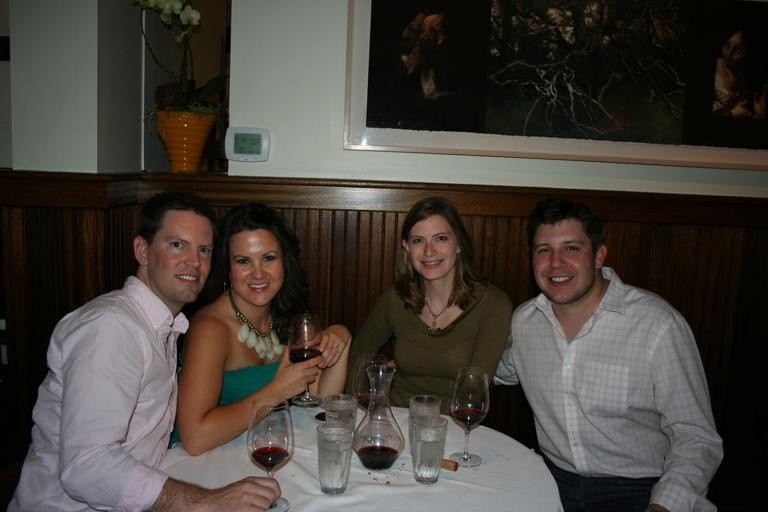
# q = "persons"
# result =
<box><xmin>713</xmin><ymin>27</ymin><xmax>767</xmax><ymax>119</ymax></box>
<box><xmin>4</xmin><ymin>189</ymin><xmax>281</xmax><ymax>512</ymax></box>
<box><xmin>383</xmin><ymin>196</ymin><xmax>725</xmax><ymax>512</ymax></box>
<box><xmin>344</xmin><ymin>197</ymin><xmax>513</xmax><ymax>418</ymax></box>
<box><xmin>168</xmin><ymin>202</ymin><xmax>353</xmax><ymax>456</ymax></box>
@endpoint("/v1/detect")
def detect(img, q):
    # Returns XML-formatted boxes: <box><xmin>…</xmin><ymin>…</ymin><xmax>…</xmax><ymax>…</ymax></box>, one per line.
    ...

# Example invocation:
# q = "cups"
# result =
<box><xmin>315</xmin><ymin>421</ymin><xmax>355</xmax><ymax>495</ymax></box>
<box><xmin>322</xmin><ymin>391</ymin><xmax>359</xmax><ymax>425</ymax></box>
<box><xmin>407</xmin><ymin>393</ymin><xmax>442</xmax><ymax>443</ymax></box>
<box><xmin>355</xmin><ymin>365</ymin><xmax>393</xmax><ymax>416</ymax></box>
<box><xmin>410</xmin><ymin>414</ymin><xmax>447</xmax><ymax>484</ymax></box>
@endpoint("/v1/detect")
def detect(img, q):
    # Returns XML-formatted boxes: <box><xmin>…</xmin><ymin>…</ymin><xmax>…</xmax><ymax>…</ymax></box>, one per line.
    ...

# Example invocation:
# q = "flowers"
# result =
<box><xmin>129</xmin><ymin>0</ymin><xmax>229</xmax><ymax>142</ymax></box>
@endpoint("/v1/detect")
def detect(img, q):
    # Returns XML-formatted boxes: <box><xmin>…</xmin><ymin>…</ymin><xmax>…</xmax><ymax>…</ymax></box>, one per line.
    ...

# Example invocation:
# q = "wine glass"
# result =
<box><xmin>447</xmin><ymin>364</ymin><xmax>491</xmax><ymax>469</ymax></box>
<box><xmin>285</xmin><ymin>308</ymin><xmax>325</xmax><ymax>407</ymax></box>
<box><xmin>244</xmin><ymin>396</ymin><xmax>295</xmax><ymax>512</ymax></box>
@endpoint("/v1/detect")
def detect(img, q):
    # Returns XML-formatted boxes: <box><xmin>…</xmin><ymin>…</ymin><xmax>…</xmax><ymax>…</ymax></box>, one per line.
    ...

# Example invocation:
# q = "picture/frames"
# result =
<box><xmin>343</xmin><ymin>0</ymin><xmax>768</xmax><ymax>172</ymax></box>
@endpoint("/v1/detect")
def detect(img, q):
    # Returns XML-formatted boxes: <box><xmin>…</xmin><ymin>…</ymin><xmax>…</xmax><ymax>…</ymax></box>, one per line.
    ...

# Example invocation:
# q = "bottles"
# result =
<box><xmin>353</xmin><ymin>362</ymin><xmax>406</xmax><ymax>471</ymax></box>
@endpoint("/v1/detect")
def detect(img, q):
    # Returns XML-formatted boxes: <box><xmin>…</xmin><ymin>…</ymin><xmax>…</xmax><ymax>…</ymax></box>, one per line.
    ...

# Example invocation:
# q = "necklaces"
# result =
<box><xmin>229</xmin><ymin>287</ymin><xmax>283</xmax><ymax>361</ymax></box>
<box><xmin>424</xmin><ymin>300</ymin><xmax>450</xmax><ymax>336</ymax></box>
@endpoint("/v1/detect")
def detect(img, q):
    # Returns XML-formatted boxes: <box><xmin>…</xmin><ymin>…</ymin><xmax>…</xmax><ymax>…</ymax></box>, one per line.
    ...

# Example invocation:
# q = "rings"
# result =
<box><xmin>334</xmin><ymin>347</ymin><xmax>340</xmax><ymax>352</ymax></box>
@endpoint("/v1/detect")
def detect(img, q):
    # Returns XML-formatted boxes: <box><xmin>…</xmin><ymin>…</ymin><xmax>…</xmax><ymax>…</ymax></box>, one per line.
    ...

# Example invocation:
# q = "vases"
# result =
<box><xmin>154</xmin><ymin>110</ymin><xmax>217</xmax><ymax>173</ymax></box>
